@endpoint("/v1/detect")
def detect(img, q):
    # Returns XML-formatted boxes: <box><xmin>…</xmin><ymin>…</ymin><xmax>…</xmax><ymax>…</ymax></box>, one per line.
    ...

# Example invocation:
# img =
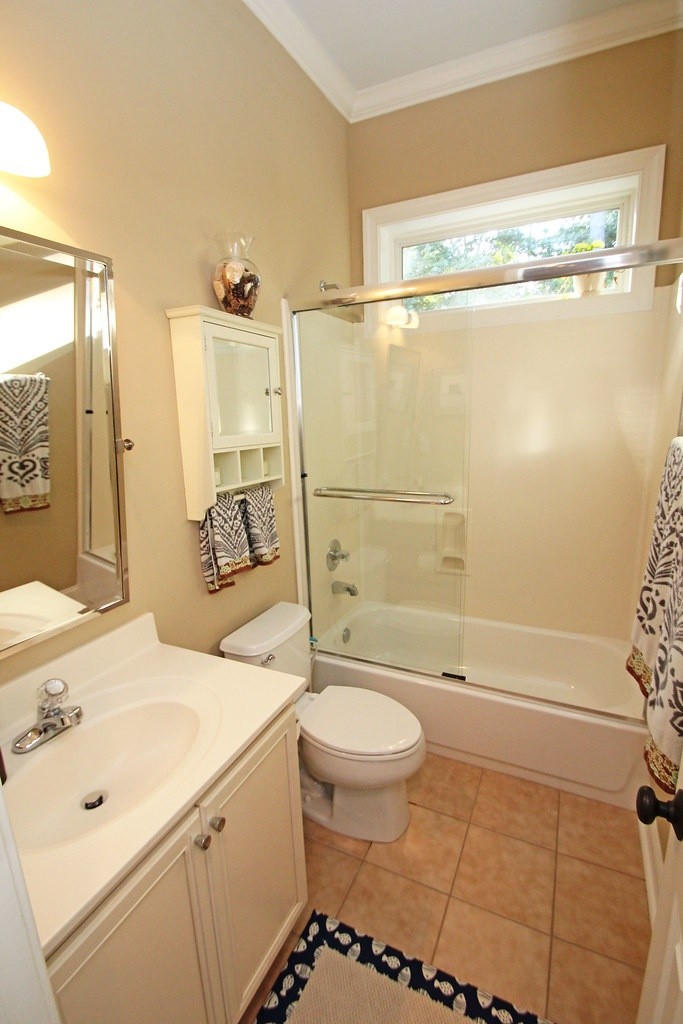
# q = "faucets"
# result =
<box><xmin>11</xmin><ymin>677</ymin><xmax>84</xmax><ymax>755</ymax></box>
<box><xmin>331</xmin><ymin>580</ymin><xmax>359</xmax><ymax>597</ymax></box>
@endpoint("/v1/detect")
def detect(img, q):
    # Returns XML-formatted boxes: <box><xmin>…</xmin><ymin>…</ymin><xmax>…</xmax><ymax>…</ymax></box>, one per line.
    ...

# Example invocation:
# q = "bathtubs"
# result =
<box><xmin>309</xmin><ymin>600</ymin><xmax>653</xmax><ymax>813</ymax></box>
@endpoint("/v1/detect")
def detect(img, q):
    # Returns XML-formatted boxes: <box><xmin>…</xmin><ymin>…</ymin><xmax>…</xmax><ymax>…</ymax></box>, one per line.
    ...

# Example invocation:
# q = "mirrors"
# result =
<box><xmin>0</xmin><ymin>225</ymin><xmax>131</xmax><ymax>661</ymax></box>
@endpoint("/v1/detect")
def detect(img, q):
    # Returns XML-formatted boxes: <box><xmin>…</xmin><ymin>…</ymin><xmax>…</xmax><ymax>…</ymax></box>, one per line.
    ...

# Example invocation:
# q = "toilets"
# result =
<box><xmin>218</xmin><ymin>600</ymin><xmax>427</xmax><ymax>844</ymax></box>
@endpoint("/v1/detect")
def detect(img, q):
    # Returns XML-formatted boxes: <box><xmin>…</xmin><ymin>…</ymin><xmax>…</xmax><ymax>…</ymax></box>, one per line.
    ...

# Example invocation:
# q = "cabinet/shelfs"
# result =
<box><xmin>165</xmin><ymin>305</ymin><xmax>286</xmax><ymax>522</ymax></box>
<box><xmin>45</xmin><ymin>703</ymin><xmax>307</xmax><ymax>1024</ymax></box>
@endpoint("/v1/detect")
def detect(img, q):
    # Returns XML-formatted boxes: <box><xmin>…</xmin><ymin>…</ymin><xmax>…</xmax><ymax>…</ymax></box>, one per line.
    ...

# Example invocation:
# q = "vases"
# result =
<box><xmin>213</xmin><ymin>230</ymin><xmax>260</xmax><ymax>318</ymax></box>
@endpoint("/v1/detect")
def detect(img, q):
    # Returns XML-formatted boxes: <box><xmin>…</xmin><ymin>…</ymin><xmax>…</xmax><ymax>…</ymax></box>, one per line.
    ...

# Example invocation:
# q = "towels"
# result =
<box><xmin>199</xmin><ymin>490</ymin><xmax>253</xmax><ymax>595</ymax></box>
<box><xmin>0</xmin><ymin>371</ymin><xmax>52</xmax><ymax>516</ymax></box>
<box><xmin>232</xmin><ymin>481</ymin><xmax>281</xmax><ymax>571</ymax></box>
<box><xmin>625</xmin><ymin>436</ymin><xmax>682</xmax><ymax>797</ymax></box>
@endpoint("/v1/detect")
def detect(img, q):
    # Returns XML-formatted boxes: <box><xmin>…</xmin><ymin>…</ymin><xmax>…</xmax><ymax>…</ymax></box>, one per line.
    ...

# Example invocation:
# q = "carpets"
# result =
<box><xmin>247</xmin><ymin>908</ymin><xmax>555</xmax><ymax>1024</ymax></box>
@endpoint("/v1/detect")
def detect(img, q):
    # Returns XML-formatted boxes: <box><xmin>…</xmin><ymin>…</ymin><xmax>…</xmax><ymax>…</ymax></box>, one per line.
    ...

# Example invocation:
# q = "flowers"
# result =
<box><xmin>214</xmin><ymin>260</ymin><xmax>260</xmax><ymax>317</ymax></box>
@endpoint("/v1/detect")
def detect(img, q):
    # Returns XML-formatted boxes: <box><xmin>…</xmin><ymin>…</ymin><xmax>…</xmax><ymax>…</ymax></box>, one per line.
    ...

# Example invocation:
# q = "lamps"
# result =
<box><xmin>0</xmin><ymin>102</ymin><xmax>51</xmax><ymax>176</ymax></box>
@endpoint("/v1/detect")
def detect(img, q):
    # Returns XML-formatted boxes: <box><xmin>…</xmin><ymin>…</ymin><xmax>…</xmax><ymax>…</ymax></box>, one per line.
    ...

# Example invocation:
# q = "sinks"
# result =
<box><xmin>1</xmin><ymin>673</ymin><xmax>226</xmax><ymax>856</ymax></box>
<box><xmin>1</xmin><ymin>611</ymin><xmax>52</xmax><ymax>649</ymax></box>
